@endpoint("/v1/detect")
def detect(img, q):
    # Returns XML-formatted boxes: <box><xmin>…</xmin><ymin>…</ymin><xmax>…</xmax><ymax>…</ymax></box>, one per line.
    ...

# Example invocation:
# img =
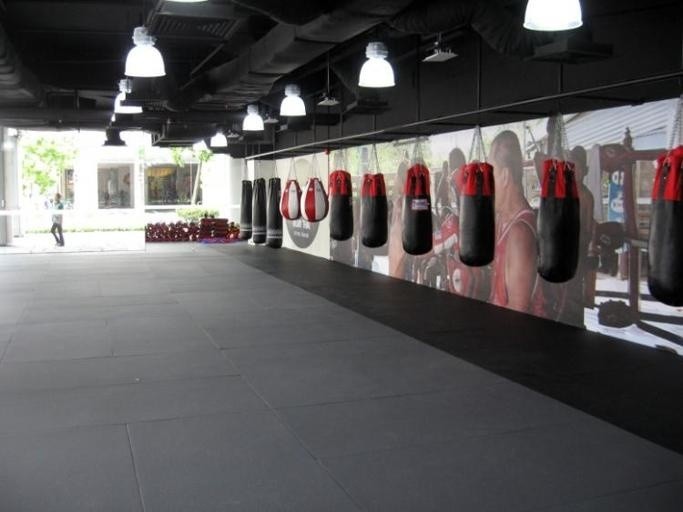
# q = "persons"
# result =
<box><xmin>45</xmin><ymin>193</ymin><xmax>64</xmax><ymax>246</ymax></box>
<box><xmin>329</xmin><ymin>130</ymin><xmax>599</xmax><ymax>327</ymax></box>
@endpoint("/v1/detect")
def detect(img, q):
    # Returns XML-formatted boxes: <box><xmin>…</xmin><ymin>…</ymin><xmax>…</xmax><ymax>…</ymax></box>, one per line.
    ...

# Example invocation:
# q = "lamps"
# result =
<box><xmin>104</xmin><ymin>126</ymin><xmax>126</xmax><ymax>148</ymax></box>
<box><xmin>124</xmin><ymin>27</ymin><xmax>166</xmax><ymax>77</ymax></box>
<box><xmin>280</xmin><ymin>84</ymin><xmax>307</xmax><ymax>116</ymax></box>
<box><xmin>263</xmin><ymin>107</ymin><xmax>279</xmax><ymax>126</ymax></box>
<box><xmin>523</xmin><ymin>0</ymin><xmax>583</xmax><ymax>32</ymax></box>
<box><xmin>241</xmin><ymin>105</ymin><xmax>265</xmax><ymax>131</ymax></box>
<box><xmin>423</xmin><ymin>30</ymin><xmax>460</xmax><ymax>64</ymax></box>
<box><xmin>357</xmin><ymin>42</ymin><xmax>396</xmax><ymax>89</ymax></box>
<box><xmin>317</xmin><ymin>49</ymin><xmax>341</xmax><ymax>107</ymax></box>
<box><xmin>208</xmin><ymin>132</ymin><xmax>231</xmax><ymax>150</ymax></box>
<box><xmin>114</xmin><ymin>79</ymin><xmax>144</xmax><ymax>114</ymax></box>
